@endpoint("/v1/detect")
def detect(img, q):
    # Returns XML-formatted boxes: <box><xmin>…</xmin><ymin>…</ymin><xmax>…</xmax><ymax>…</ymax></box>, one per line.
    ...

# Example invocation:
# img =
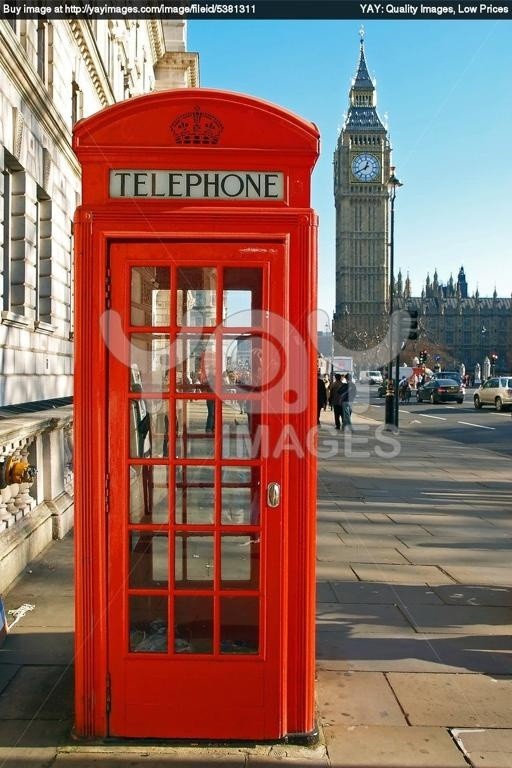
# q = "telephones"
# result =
<box><xmin>130</xmin><ymin>363</ymin><xmax>153</xmax><ymax>458</ymax></box>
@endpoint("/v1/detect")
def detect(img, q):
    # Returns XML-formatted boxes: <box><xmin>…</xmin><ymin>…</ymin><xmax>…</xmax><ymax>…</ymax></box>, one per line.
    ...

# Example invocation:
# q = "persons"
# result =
<box><xmin>397</xmin><ymin>376</ymin><xmax>413</xmax><ymax>402</ymax></box>
<box><xmin>462</xmin><ymin>373</ymin><xmax>470</xmax><ymax>401</ymax></box>
<box><xmin>383</xmin><ymin>373</ymin><xmax>388</xmax><ymax>396</ymax></box>
<box><xmin>180</xmin><ymin>364</ymin><xmax>357</xmax><ymax>437</ymax></box>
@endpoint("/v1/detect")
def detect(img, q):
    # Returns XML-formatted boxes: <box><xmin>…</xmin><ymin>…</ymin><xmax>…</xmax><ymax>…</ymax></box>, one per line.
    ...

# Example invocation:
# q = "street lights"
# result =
<box><xmin>491</xmin><ymin>353</ymin><xmax>498</xmax><ymax>378</ymax></box>
<box><xmin>479</xmin><ymin>325</ymin><xmax>486</xmax><ymax>380</ymax></box>
<box><xmin>379</xmin><ymin>165</ymin><xmax>404</xmax><ymax>432</ymax></box>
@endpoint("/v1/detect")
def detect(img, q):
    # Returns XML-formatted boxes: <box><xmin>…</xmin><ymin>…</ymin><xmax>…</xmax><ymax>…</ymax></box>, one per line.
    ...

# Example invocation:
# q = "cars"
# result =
<box><xmin>356</xmin><ymin>365</ymin><xmax>512</xmax><ymax>412</ymax></box>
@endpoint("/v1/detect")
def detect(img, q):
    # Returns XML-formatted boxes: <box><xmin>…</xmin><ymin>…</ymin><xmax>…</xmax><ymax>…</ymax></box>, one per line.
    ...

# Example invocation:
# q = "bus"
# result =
<box><xmin>316</xmin><ymin>356</ymin><xmax>354</xmax><ymax>391</ymax></box>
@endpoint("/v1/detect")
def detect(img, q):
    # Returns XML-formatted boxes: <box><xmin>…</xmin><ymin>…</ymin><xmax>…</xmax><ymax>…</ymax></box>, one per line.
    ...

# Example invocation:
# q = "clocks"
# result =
<box><xmin>351</xmin><ymin>151</ymin><xmax>382</xmax><ymax>182</ymax></box>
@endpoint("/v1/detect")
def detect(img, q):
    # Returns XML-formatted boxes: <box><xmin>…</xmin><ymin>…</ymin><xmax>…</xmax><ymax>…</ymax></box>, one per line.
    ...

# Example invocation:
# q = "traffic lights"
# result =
<box><xmin>423</xmin><ymin>349</ymin><xmax>427</xmax><ymax>363</ymax></box>
<box><xmin>397</xmin><ymin>307</ymin><xmax>417</xmax><ymax>340</ymax></box>
<box><xmin>419</xmin><ymin>350</ymin><xmax>423</xmax><ymax>364</ymax></box>
